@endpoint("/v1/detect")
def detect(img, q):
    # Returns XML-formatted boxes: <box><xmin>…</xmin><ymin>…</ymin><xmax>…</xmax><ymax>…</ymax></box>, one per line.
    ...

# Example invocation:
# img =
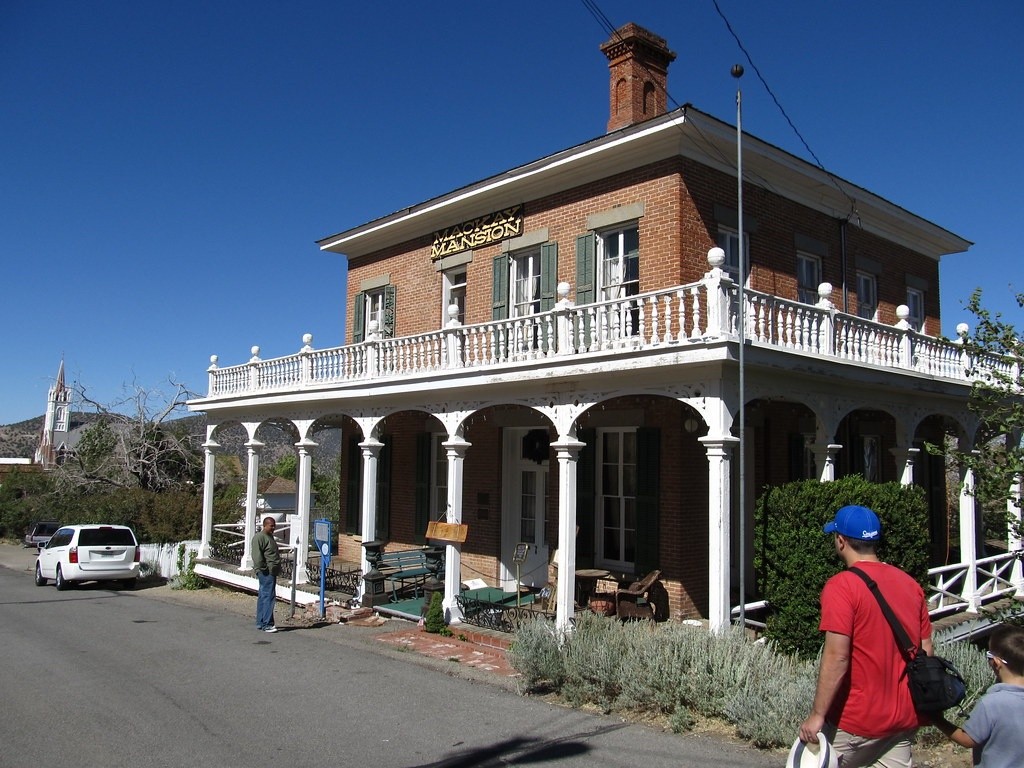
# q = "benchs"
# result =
<box><xmin>380</xmin><ymin>548</ymin><xmax>435</xmax><ymax>603</ymax></box>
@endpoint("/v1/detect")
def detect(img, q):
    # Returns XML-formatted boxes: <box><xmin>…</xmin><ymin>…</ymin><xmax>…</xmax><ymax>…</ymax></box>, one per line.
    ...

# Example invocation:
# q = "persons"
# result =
<box><xmin>800</xmin><ymin>506</ymin><xmax>935</xmax><ymax>768</ymax></box>
<box><xmin>252</xmin><ymin>516</ymin><xmax>282</xmax><ymax>634</ymax></box>
<box><xmin>926</xmin><ymin>621</ymin><xmax>1024</xmax><ymax>768</ymax></box>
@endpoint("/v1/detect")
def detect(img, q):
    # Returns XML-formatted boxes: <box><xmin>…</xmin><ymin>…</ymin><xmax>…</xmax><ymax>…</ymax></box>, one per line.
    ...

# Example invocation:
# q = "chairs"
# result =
<box><xmin>615</xmin><ymin>569</ymin><xmax>662</xmax><ymax>623</ymax></box>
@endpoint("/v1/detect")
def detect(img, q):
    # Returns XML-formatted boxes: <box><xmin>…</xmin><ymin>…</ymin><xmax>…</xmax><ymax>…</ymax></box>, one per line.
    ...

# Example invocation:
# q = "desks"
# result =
<box><xmin>575</xmin><ymin>569</ymin><xmax>612</xmax><ymax>617</ymax></box>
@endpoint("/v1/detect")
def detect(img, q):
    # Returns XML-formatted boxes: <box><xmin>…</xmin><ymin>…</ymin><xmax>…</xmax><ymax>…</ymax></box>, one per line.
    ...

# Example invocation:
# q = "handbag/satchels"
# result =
<box><xmin>908</xmin><ymin>655</ymin><xmax>968</xmax><ymax>713</ymax></box>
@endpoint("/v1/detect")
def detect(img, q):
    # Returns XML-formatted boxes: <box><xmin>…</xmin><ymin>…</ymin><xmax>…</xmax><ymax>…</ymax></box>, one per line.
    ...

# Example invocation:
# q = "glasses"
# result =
<box><xmin>987</xmin><ymin>651</ymin><xmax>1007</xmax><ymax>663</ymax></box>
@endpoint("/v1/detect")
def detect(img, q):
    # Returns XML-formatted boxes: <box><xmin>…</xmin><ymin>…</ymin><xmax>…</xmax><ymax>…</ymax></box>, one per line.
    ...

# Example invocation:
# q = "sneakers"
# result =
<box><xmin>262</xmin><ymin>625</ymin><xmax>277</xmax><ymax>632</ymax></box>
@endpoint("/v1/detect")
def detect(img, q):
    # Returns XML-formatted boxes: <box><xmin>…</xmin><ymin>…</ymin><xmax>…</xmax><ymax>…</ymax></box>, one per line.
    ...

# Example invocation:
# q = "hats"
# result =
<box><xmin>786</xmin><ymin>732</ymin><xmax>838</xmax><ymax>768</ymax></box>
<box><xmin>824</xmin><ymin>505</ymin><xmax>880</xmax><ymax>540</ymax></box>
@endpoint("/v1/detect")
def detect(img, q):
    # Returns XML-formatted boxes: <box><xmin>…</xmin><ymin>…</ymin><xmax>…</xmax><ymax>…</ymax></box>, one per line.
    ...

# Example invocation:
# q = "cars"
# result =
<box><xmin>34</xmin><ymin>524</ymin><xmax>140</xmax><ymax>590</ymax></box>
